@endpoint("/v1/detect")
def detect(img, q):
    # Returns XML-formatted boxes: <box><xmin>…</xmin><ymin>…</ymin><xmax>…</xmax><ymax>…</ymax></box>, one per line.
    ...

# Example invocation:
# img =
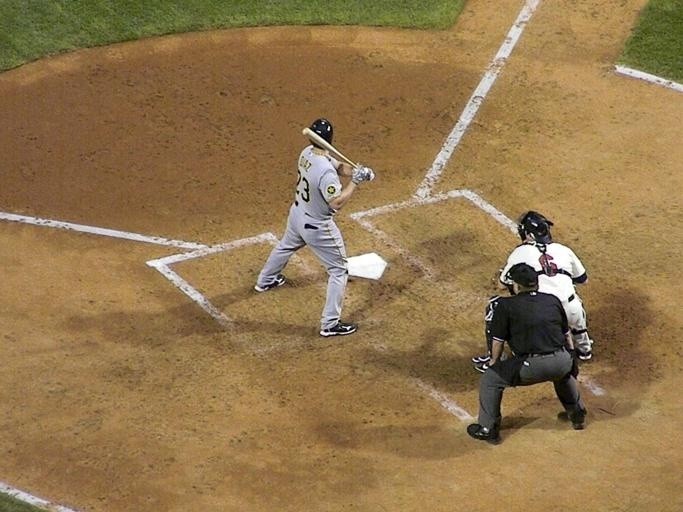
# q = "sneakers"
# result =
<box><xmin>475</xmin><ymin>360</ymin><xmax>490</xmax><ymax>374</ymax></box>
<box><xmin>468</xmin><ymin>424</ymin><xmax>501</xmax><ymax>445</ymax></box>
<box><xmin>576</xmin><ymin>349</ymin><xmax>592</xmax><ymax>360</ymax></box>
<box><xmin>571</xmin><ymin>407</ymin><xmax>586</xmax><ymax>429</ymax></box>
<box><xmin>320</xmin><ymin>320</ymin><xmax>357</xmax><ymax>337</ymax></box>
<box><xmin>255</xmin><ymin>274</ymin><xmax>285</xmax><ymax>292</ymax></box>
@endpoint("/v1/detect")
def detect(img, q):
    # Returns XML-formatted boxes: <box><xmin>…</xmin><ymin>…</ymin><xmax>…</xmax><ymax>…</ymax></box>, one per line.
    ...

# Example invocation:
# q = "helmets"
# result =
<box><xmin>505</xmin><ymin>264</ymin><xmax>538</xmax><ymax>295</ymax></box>
<box><xmin>518</xmin><ymin>211</ymin><xmax>553</xmax><ymax>244</ymax></box>
<box><xmin>309</xmin><ymin>119</ymin><xmax>332</xmax><ymax>149</ymax></box>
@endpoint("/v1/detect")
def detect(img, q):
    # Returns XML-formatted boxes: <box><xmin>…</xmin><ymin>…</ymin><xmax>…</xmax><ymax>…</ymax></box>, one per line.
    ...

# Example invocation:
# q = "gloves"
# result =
<box><xmin>352</xmin><ymin>163</ymin><xmax>375</xmax><ymax>185</ymax></box>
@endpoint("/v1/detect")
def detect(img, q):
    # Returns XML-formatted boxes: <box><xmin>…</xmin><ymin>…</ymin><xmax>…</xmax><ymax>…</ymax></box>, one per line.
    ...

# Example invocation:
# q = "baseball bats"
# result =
<box><xmin>301</xmin><ymin>128</ymin><xmax>358</xmax><ymax>168</ymax></box>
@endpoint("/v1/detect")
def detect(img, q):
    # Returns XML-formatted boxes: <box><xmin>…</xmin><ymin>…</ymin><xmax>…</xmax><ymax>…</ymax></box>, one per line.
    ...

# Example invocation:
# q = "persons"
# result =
<box><xmin>465</xmin><ymin>261</ymin><xmax>588</xmax><ymax>445</ymax></box>
<box><xmin>471</xmin><ymin>209</ymin><xmax>594</xmax><ymax>373</ymax></box>
<box><xmin>253</xmin><ymin>117</ymin><xmax>376</xmax><ymax>338</ymax></box>
